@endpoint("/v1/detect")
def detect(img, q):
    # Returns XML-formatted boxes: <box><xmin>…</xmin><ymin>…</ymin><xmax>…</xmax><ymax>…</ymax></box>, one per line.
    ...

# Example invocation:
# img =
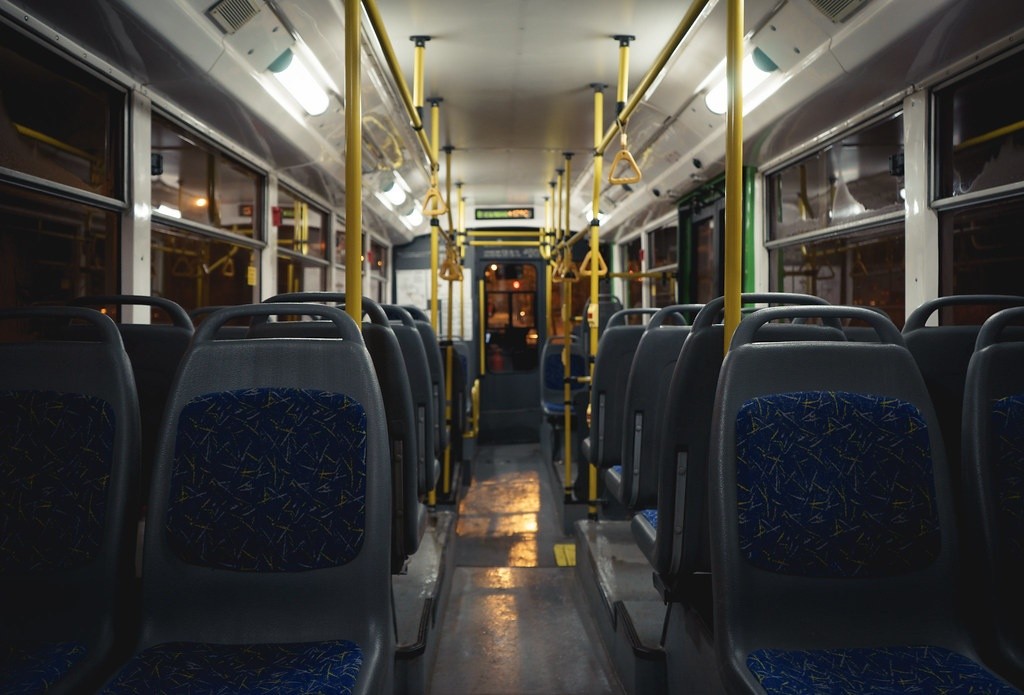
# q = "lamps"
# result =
<box><xmin>586</xmin><ymin>198</ymin><xmax>616</xmax><ymax>223</ymax></box>
<box><xmin>382</xmin><ymin>178</ymin><xmax>407</xmax><ymax>206</ymax></box>
<box><xmin>269</xmin><ymin>47</ymin><xmax>330</xmax><ymax>117</ymax></box>
<box><xmin>704</xmin><ymin>46</ymin><xmax>777</xmax><ymax>116</ymax></box>
<box><xmin>400</xmin><ymin>204</ymin><xmax>423</xmax><ymax>228</ymax></box>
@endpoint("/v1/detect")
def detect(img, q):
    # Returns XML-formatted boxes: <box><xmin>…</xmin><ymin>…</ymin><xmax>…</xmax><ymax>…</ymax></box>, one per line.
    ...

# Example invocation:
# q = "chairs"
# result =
<box><xmin>0</xmin><ymin>291</ymin><xmax>475</xmax><ymax>695</ymax></box>
<box><xmin>536</xmin><ymin>295</ymin><xmax>1024</xmax><ymax>695</ymax></box>
